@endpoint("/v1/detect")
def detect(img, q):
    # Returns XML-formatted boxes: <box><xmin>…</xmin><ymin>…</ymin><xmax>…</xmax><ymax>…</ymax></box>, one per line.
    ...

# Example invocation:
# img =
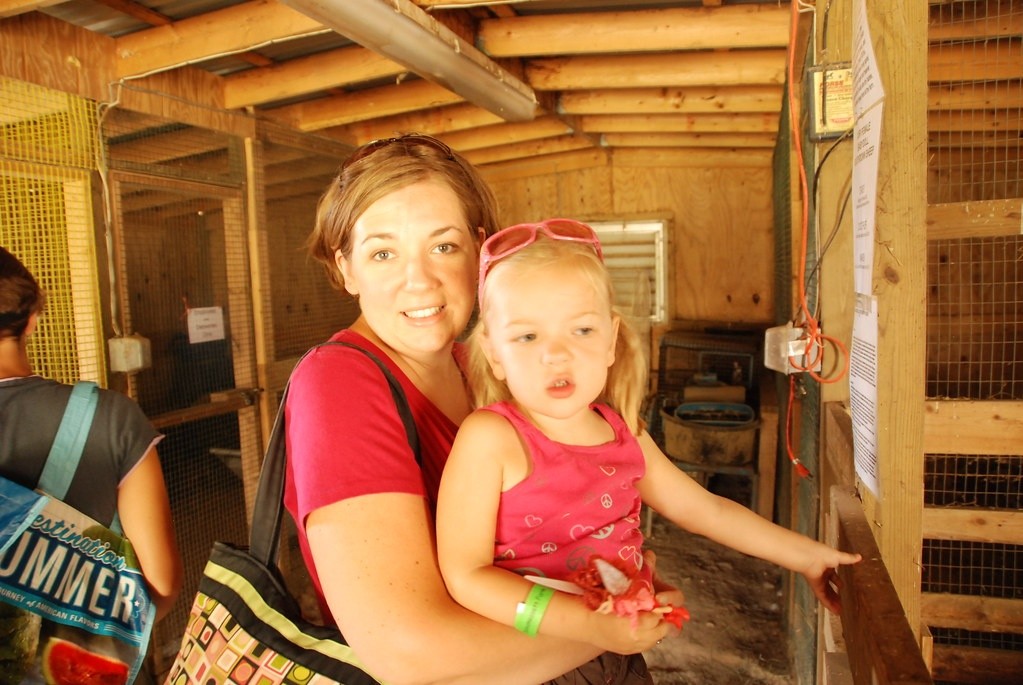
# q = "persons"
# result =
<box><xmin>284</xmin><ymin>129</ymin><xmax>861</xmax><ymax>685</ymax></box>
<box><xmin>0</xmin><ymin>247</ymin><xmax>184</xmax><ymax>685</ymax></box>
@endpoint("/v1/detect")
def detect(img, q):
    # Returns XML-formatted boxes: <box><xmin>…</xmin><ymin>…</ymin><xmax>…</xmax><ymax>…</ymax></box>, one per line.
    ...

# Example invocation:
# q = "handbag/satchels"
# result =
<box><xmin>162</xmin><ymin>338</ymin><xmax>420</xmax><ymax>685</ymax></box>
<box><xmin>0</xmin><ymin>382</ymin><xmax>155</xmax><ymax>685</ymax></box>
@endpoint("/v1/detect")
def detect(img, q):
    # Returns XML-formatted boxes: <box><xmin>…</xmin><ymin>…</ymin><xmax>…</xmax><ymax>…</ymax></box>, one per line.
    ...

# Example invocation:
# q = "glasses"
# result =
<box><xmin>339</xmin><ymin>134</ymin><xmax>455</xmax><ymax>195</ymax></box>
<box><xmin>477</xmin><ymin>219</ymin><xmax>603</xmax><ymax>317</ymax></box>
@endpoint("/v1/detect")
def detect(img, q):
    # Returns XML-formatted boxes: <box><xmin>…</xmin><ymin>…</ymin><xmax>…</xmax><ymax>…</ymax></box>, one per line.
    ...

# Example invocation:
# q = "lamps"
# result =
<box><xmin>281</xmin><ymin>0</ymin><xmax>540</xmax><ymax>121</ymax></box>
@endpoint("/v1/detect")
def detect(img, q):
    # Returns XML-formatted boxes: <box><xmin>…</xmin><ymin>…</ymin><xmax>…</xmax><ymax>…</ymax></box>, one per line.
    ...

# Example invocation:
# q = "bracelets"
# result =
<box><xmin>514</xmin><ymin>583</ymin><xmax>557</xmax><ymax>639</ymax></box>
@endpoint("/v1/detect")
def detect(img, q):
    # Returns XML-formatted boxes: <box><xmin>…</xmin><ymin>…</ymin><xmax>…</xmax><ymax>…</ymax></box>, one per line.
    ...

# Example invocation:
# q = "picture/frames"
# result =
<box><xmin>807</xmin><ymin>62</ymin><xmax>854</xmax><ymax>141</ymax></box>
